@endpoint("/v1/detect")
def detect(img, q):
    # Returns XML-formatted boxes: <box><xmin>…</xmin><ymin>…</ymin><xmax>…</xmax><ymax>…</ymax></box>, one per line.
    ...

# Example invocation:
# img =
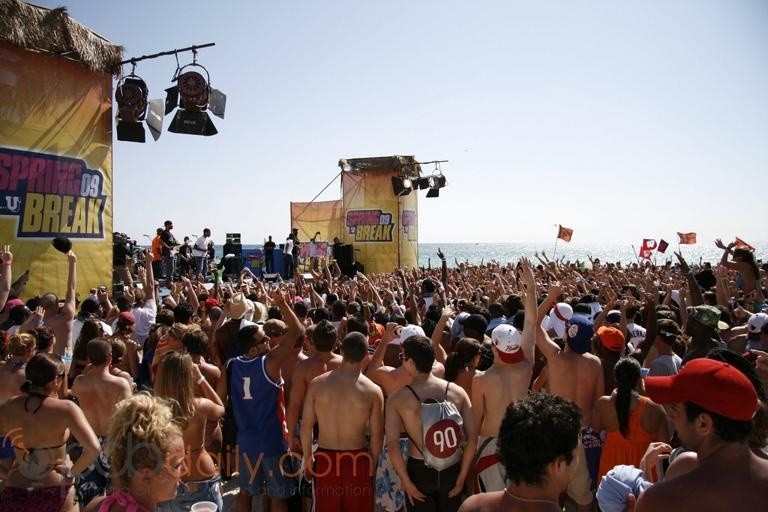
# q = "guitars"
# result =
<box><xmin>169</xmin><ymin>239</ymin><xmax>192</xmax><ymax>258</ymax></box>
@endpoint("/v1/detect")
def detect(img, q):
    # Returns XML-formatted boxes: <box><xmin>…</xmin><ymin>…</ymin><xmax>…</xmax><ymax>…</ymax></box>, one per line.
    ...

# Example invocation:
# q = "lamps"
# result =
<box><xmin>386</xmin><ymin>157</ymin><xmax>451</xmax><ymax>204</ymax></box>
<box><xmin>106</xmin><ymin>32</ymin><xmax>230</xmax><ymax>145</ymax></box>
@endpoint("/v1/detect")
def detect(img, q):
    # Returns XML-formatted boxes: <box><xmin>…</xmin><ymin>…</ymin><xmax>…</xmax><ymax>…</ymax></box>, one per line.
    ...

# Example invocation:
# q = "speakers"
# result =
<box><xmin>333</xmin><ymin>243</ymin><xmax>354</xmax><ymax>272</ymax></box>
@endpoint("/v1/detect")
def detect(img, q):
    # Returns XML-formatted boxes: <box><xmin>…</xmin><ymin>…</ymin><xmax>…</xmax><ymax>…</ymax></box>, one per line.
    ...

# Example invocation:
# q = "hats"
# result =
<box><xmin>567</xmin><ymin>315</ymin><xmax>593</xmax><ymax>354</ymax></box>
<box><xmin>597</xmin><ymin>326</ymin><xmax>626</xmax><ymax>351</ymax></box>
<box><xmin>418</xmin><ymin>279</ymin><xmax>436</xmax><ymax>291</ymax></box>
<box><xmin>549</xmin><ymin>301</ymin><xmax>574</xmax><ymax>342</ymax></box>
<box><xmin>52</xmin><ymin>236</ymin><xmax>72</xmax><ymax>254</ymax></box>
<box><xmin>643</xmin><ymin>357</ymin><xmax>763</xmax><ymax>421</ymax></box>
<box><xmin>492</xmin><ymin>324</ymin><xmax>524</xmax><ymax>364</ymax></box>
<box><xmin>223</xmin><ymin>292</ymin><xmax>253</xmax><ymax>320</ymax></box>
<box><xmin>747</xmin><ymin>312</ymin><xmax>768</xmax><ymax>333</ymax></box>
<box><xmin>463</xmin><ymin>313</ymin><xmax>489</xmax><ymax>334</ymax></box>
<box><xmin>400</xmin><ymin>323</ymin><xmax>427</xmax><ymax>342</ymax></box>
<box><xmin>118</xmin><ymin>311</ymin><xmax>136</xmax><ymax>323</ymax></box>
<box><xmin>685</xmin><ymin>303</ymin><xmax>722</xmax><ymax>330</ymax></box>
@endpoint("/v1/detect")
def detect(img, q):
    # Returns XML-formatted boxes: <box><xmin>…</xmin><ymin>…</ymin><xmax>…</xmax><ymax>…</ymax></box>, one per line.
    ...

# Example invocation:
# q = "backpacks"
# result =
<box><xmin>402</xmin><ymin>379</ymin><xmax>465</xmax><ymax>471</ymax></box>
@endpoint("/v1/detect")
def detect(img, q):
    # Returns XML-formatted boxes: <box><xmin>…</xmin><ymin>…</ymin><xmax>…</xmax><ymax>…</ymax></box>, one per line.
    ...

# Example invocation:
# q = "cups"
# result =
<box><xmin>60</xmin><ymin>356</ymin><xmax>72</xmax><ymax>374</ymax></box>
<box><xmin>191</xmin><ymin>500</ymin><xmax>218</xmax><ymax>511</ymax></box>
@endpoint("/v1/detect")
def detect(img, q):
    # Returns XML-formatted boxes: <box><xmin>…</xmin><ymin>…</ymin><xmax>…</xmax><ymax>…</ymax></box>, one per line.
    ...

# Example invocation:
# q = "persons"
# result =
<box><xmin>0</xmin><ymin>219</ymin><xmax>768</xmax><ymax>512</ymax></box>
